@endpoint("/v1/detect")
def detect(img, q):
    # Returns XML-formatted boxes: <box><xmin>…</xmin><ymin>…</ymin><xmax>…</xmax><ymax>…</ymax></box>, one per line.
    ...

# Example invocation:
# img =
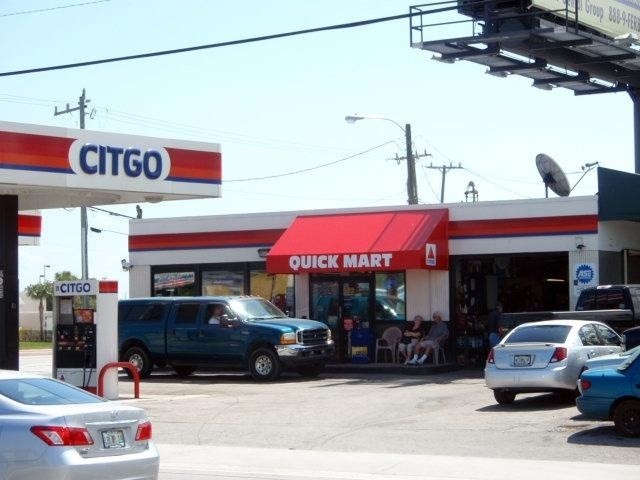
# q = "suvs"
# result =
<box><xmin>118</xmin><ymin>295</ymin><xmax>334</xmax><ymax>382</ymax></box>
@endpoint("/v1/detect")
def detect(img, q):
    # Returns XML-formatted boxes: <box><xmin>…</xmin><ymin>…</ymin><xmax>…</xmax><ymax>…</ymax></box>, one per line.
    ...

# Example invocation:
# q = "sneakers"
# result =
<box><xmin>406</xmin><ymin>358</ymin><xmax>417</xmax><ymax>364</ymax></box>
<box><xmin>418</xmin><ymin>359</ymin><xmax>424</xmax><ymax>364</ymax></box>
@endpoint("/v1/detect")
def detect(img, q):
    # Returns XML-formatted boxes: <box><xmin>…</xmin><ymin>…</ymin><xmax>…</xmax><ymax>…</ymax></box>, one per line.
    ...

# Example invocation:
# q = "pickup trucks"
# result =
<box><xmin>496</xmin><ymin>284</ymin><xmax>640</xmax><ymax>337</ymax></box>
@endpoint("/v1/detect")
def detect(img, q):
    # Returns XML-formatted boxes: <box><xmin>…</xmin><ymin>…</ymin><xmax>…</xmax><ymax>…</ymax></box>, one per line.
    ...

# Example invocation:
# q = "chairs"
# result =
<box><xmin>412</xmin><ymin>327</ymin><xmax>449</xmax><ymax>365</ymax></box>
<box><xmin>375</xmin><ymin>326</ymin><xmax>402</xmax><ymax>362</ymax></box>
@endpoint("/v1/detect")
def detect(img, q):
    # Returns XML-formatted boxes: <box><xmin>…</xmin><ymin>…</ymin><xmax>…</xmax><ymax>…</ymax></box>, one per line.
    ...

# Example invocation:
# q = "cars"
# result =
<box><xmin>484</xmin><ymin>320</ymin><xmax>621</xmax><ymax>405</ymax></box>
<box><xmin>0</xmin><ymin>370</ymin><xmax>159</xmax><ymax>479</ymax></box>
<box><xmin>583</xmin><ymin>343</ymin><xmax>640</xmax><ymax>369</ymax></box>
<box><xmin>620</xmin><ymin>326</ymin><xmax>640</xmax><ymax>352</ymax></box>
<box><xmin>576</xmin><ymin>350</ymin><xmax>640</xmax><ymax>437</ymax></box>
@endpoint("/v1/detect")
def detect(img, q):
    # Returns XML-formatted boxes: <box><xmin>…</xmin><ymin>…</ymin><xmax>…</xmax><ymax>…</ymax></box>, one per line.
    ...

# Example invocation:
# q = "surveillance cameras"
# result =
<box><xmin>121</xmin><ymin>259</ymin><xmax>133</xmax><ymax>271</ymax></box>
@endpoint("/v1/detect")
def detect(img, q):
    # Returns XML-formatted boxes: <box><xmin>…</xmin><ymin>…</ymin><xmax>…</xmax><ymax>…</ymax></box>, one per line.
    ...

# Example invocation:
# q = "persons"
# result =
<box><xmin>486</xmin><ymin>301</ymin><xmax>504</xmax><ymax>330</ymax></box>
<box><xmin>207</xmin><ymin>304</ymin><xmax>226</xmax><ymax>326</ymax></box>
<box><xmin>406</xmin><ymin>311</ymin><xmax>450</xmax><ymax>365</ymax></box>
<box><xmin>398</xmin><ymin>315</ymin><xmax>424</xmax><ymax>366</ymax></box>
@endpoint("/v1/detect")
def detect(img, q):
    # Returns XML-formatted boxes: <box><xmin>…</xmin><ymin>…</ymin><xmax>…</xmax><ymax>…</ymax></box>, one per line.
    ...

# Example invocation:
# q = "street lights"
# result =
<box><xmin>44</xmin><ymin>265</ymin><xmax>50</xmax><ymax>282</ymax></box>
<box><xmin>39</xmin><ymin>274</ymin><xmax>45</xmax><ymax>285</ymax></box>
<box><xmin>344</xmin><ymin>115</ymin><xmax>419</xmax><ymax>204</ymax></box>
